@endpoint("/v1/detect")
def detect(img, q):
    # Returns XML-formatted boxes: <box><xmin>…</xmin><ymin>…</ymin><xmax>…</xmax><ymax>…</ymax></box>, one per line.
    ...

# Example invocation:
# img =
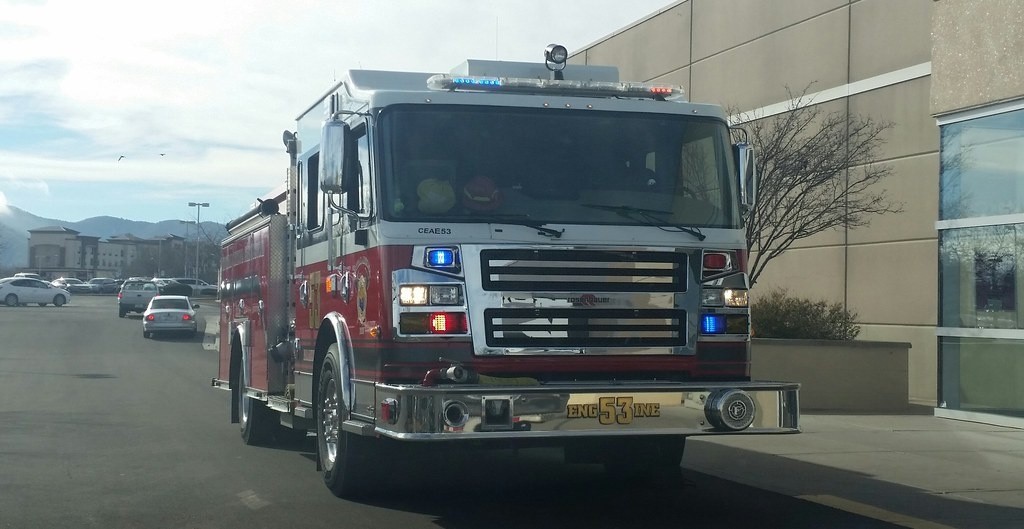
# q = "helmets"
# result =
<box><xmin>416</xmin><ymin>178</ymin><xmax>455</xmax><ymax>214</ymax></box>
<box><xmin>463</xmin><ymin>175</ymin><xmax>502</xmax><ymax>211</ymax></box>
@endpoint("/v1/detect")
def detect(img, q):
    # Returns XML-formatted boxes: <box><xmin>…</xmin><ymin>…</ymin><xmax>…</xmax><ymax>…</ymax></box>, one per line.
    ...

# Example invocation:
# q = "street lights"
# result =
<box><xmin>180</xmin><ymin>220</ymin><xmax>195</xmax><ymax>278</ymax></box>
<box><xmin>189</xmin><ymin>202</ymin><xmax>209</xmax><ymax>294</ymax></box>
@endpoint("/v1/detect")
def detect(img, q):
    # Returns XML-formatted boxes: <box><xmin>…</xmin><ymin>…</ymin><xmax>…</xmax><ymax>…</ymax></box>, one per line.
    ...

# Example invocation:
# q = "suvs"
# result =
<box><xmin>118</xmin><ymin>279</ymin><xmax>161</xmax><ymax>317</ymax></box>
<box><xmin>14</xmin><ymin>273</ymin><xmax>51</xmax><ymax>285</ymax></box>
<box><xmin>178</xmin><ymin>278</ymin><xmax>218</xmax><ymax>295</ymax></box>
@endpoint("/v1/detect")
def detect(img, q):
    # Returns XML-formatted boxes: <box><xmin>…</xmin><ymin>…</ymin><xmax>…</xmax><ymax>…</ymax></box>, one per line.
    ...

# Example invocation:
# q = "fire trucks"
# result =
<box><xmin>212</xmin><ymin>44</ymin><xmax>803</xmax><ymax>502</ymax></box>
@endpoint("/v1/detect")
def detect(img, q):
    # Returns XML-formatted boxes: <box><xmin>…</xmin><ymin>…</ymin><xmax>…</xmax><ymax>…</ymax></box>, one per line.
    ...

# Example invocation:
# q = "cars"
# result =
<box><xmin>142</xmin><ymin>296</ymin><xmax>200</xmax><ymax>339</ymax></box>
<box><xmin>87</xmin><ymin>278</ymin><xmax>117</xmax><ymax>293</ymax></box>
<box><xmin>51</xmin><ymin>277</ymin><xmax>89</xmax><ymax>294</ymax></box>
<box><xmin>0</xmin><ymin>277</ymin><xmax>71</xmax><ymax>306</ymax></box>
<box><xmin>115</xmin><ymin>279</ymin><xmax>126</xmax><ymax>292</ymax></box>
<box><xmin>151</xmin><ymin>278</ymin><xmax>193</xmax><ymax>296</ymax></box>
